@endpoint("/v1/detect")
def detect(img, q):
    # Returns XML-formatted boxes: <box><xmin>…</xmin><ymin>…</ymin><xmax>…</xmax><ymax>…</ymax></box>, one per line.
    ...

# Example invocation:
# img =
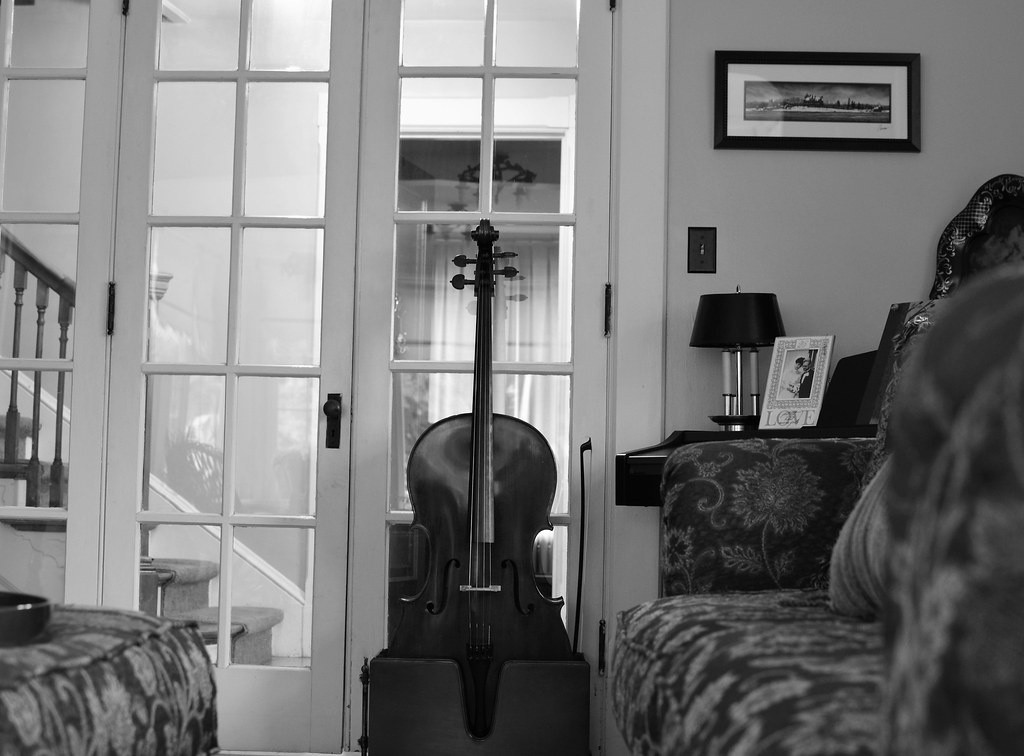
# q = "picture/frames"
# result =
<box><xmin>714</xmin><ymin>51</ymin><xmax>922</xmax><ymax>153</ymax></box>
<box><xmin>759</xmin><ymin>336</ymin><xmax>836</xmax><ymax>430</ymax></box>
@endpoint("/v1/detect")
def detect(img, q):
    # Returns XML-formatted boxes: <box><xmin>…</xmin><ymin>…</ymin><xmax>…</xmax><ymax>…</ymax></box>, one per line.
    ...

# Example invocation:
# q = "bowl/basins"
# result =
<box><xmin>0</xmin><ymin>590</ymin><xmax>52</xmax><ymax>647</ymax></box>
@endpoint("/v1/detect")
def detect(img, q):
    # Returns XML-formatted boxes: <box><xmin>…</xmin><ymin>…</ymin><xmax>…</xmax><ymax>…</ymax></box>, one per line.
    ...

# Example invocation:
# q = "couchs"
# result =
<box><xmin>610</xmin><ymin>269</ymin><xmax>1024</xmax><ymax>756</ymax></box>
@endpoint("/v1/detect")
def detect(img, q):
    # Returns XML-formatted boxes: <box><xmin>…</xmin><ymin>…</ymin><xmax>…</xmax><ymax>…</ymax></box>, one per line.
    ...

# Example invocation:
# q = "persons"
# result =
<box><xmin>799</xmin><ymin>359</ymin><xmax>814</xmax><ymax>398</ymax></box>
<box><xmin>780</xmin><ymin>357</ymin><xmax>803</xmax><ymax>398</ymax></box>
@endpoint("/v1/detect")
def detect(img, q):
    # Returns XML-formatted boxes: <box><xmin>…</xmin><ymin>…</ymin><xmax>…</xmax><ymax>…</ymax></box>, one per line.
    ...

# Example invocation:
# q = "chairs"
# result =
<box><xmin>0</xmin><ymin>603</ymin><xmax>222</xmax><ymax>756</ymax></box>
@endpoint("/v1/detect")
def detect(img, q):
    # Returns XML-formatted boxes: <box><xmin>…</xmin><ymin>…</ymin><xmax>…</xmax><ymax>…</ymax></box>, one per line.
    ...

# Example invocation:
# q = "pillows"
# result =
<box><xmin>827</xmin><ymin>453</ymin><xmax>893</xmax><ymax>617</ymax></box>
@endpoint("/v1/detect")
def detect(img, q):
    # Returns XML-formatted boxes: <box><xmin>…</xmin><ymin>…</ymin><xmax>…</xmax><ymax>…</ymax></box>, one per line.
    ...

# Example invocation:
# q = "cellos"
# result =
<box><xmin>384</xmin><ymin>218</ymin><xmax>574</xmax><ymax>738</ymax></box>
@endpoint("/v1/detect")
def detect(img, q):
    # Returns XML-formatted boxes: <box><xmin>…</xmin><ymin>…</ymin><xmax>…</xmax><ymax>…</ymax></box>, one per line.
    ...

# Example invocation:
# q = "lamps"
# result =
<box><xmin>689</xmin><ymin>284</ymin><xmax>786</xmax><ymax>431</ymax></box>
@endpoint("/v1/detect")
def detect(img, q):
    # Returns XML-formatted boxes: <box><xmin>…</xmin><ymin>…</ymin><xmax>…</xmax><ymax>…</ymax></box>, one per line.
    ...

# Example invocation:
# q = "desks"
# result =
<box><xmin>615</xmin><ymin>424</ymin><xmax>882</xmax><ymax>507</ymax></box>
